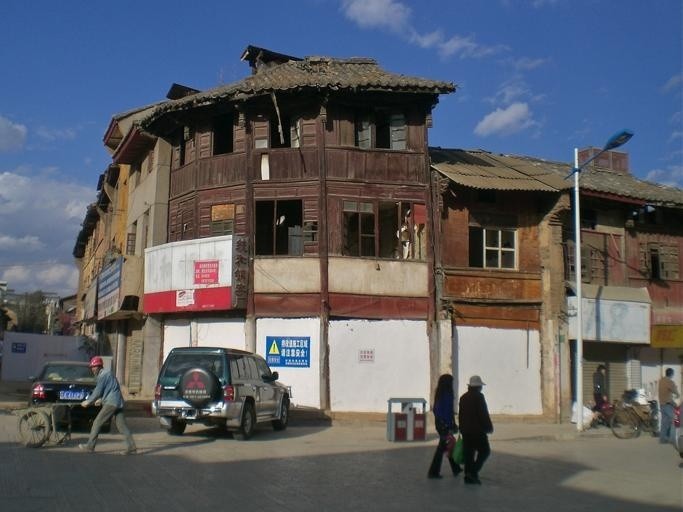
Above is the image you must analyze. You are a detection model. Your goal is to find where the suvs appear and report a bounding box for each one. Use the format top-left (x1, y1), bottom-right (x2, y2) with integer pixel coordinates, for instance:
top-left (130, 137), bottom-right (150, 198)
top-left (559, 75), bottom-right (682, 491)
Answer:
top-left (150, 346), bottom-right (291, 442)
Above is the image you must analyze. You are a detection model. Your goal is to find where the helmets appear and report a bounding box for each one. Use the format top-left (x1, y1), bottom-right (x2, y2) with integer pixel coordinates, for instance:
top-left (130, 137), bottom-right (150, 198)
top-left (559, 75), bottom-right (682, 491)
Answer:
top-left (89, 357), bottom-right (103, 366)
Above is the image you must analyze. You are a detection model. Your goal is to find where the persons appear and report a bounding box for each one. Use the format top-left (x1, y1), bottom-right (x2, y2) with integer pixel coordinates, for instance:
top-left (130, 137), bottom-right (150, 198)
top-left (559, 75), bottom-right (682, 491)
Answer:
top-left (658, 368), bottom-right (679, 444)
top-left (458, 374), bottom-right (493, 484)
top-left (426, 374), bottom-right (464, 478)
top-left (78, 356), bottom-right (137, 457)
top-left (590, 364), bottom-right (608, 412)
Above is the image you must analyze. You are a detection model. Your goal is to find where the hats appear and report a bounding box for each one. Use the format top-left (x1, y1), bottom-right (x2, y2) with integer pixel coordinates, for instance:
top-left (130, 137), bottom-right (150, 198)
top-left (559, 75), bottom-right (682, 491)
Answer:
top-left (469, 375), bottom-right (486, 387)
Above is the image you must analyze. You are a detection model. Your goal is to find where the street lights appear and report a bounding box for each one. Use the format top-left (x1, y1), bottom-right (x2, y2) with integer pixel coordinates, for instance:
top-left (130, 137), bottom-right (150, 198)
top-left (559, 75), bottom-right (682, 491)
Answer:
top-left (563, 129), bottom-right (634, 432)
top-left (41, 298), bottom-right (62, 362)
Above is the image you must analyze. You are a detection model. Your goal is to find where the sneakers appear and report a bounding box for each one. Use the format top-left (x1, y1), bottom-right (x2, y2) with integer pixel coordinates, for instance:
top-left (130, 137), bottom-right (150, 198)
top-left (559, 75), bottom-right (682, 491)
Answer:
top-left (120, 450), bottom-right (137, 456)
top-left (79, 444), bottom-right (94, 453)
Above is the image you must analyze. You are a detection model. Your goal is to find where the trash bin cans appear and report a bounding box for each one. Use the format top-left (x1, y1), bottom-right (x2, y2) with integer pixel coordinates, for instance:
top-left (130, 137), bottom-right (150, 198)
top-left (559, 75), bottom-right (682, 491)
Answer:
top-left (388, 398), bottom-right (426, 442)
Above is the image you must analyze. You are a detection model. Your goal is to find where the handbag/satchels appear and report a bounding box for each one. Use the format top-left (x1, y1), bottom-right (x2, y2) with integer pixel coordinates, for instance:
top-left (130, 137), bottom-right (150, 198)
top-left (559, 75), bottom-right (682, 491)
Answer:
top-left (452, 439), bottom-right (466, 465)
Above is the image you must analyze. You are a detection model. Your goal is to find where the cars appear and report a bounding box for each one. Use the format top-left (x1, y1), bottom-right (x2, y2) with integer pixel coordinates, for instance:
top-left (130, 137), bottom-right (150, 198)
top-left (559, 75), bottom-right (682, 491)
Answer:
top-left (26, 361), bottom-right (112, 432)
top-left (672, 402), bottom-right (683, 458)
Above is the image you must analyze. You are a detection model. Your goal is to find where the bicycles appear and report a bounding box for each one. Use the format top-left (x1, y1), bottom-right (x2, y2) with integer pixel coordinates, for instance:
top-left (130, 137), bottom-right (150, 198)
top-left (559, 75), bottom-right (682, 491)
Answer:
top-left (609, 399), bottom-right (661, 438)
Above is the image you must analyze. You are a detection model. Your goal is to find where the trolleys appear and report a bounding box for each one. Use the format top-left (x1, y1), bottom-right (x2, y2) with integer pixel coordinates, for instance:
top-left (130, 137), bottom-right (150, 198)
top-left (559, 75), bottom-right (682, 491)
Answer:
top-left (9, 399), bottom-right (101, 448)
